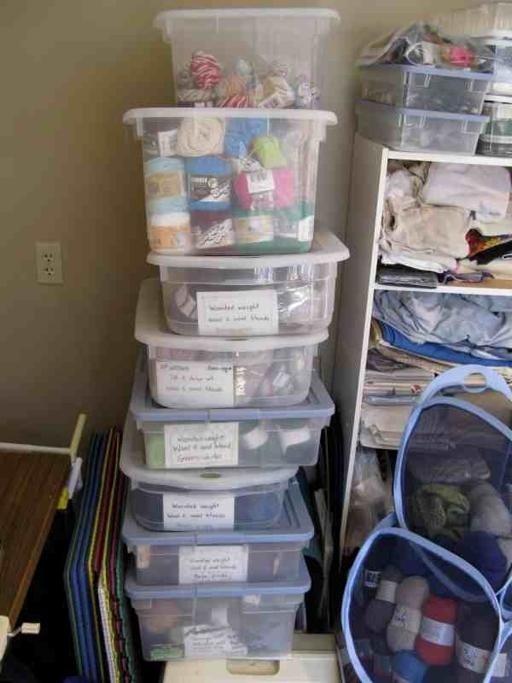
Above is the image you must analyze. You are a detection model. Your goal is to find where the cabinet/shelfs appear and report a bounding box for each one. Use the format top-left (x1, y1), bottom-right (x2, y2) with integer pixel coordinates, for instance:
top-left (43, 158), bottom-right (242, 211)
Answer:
top-left (326, 127), bottom-right (508, 630)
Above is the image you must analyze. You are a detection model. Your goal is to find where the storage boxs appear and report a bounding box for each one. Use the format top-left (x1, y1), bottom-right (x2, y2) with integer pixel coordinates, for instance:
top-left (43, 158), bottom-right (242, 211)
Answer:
top-left (150, 7), bottom-right (342, 108)
top-left (355, 6), bottom-right (511, 159)
top-left (143, 222), bottom-right (352, 335)
top-left (121, 473), bottom-right (316, 588)
top-left (124, 547), bottom-right (313, 662)
top-left (119, 103), bottom-right (341, 255)
top-left (117, 396), bottom-right (300, 534)
top-left (133, 349), bottom-right (333, 471)
top-left (133, 276), bottom-right (329, 408)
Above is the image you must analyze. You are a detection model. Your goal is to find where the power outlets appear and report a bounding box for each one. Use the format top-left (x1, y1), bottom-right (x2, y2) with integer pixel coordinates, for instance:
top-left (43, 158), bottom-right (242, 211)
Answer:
top-left (34, 238), bottom-right (66, 288)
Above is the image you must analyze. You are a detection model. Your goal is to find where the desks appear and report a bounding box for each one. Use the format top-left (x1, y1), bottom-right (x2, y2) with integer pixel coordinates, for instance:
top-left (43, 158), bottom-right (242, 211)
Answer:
top-left (1, 441), bottom-right (73, 683)
